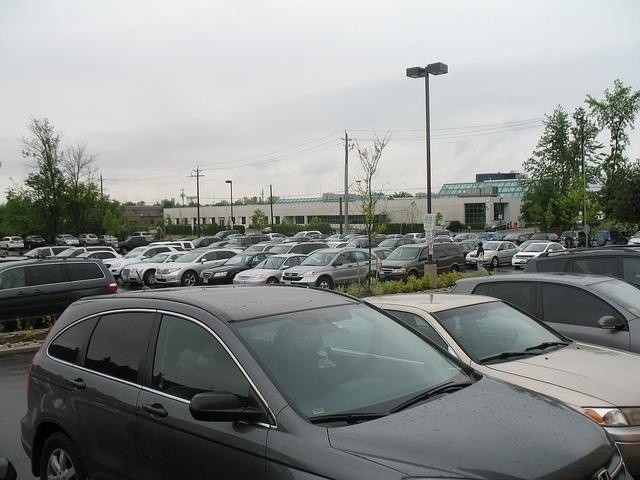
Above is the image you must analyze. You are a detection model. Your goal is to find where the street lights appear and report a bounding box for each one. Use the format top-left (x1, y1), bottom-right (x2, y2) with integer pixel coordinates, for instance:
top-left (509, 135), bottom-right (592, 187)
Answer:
top-left (225, 180), bottom-right (233, 230)
top-left (405, 62), bottom-right (450, 290)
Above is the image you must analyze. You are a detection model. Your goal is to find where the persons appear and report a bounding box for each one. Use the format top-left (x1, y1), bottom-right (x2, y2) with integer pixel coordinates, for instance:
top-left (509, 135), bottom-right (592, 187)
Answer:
top-left (468, 225), bottom-right (471, 233)
top-left (474, 241), bottom-right (485, 270)
top-left (33, 249), bottom-right (46, 259)
